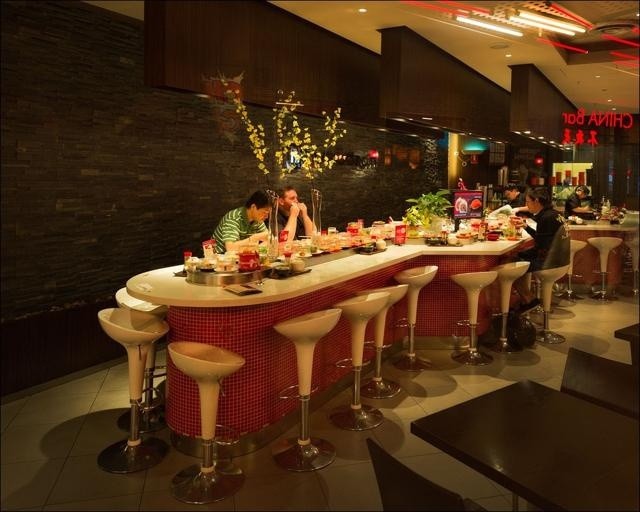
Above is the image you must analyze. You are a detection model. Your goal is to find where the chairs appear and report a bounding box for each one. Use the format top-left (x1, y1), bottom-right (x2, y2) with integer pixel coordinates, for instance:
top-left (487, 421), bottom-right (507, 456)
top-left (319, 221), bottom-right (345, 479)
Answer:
top-left (330, 292), bottom-right (384, 430)
top-left (97, 308), bottom-right (169, 474)
top-left (356, 285), bottom-right (408, 399)
top-left (395, 264), bottom-right (438, 372)
top-left (168, 340), bottom-right (246, 504)
top-left (449, 237), bottom-right (640, 365)
top-left (362, 438), bottom-right (467, 512)
top-left (115, 286), bottom-right (166, 433)
top-left (559, 348), bottom-right (638, 423)
top-left (272, 308), bottom-right (336, 472)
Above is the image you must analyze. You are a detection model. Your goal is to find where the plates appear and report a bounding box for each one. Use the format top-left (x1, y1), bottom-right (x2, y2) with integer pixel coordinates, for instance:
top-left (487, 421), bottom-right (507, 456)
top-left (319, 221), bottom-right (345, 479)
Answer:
top-left (196, 216), bottom-right (523, 279)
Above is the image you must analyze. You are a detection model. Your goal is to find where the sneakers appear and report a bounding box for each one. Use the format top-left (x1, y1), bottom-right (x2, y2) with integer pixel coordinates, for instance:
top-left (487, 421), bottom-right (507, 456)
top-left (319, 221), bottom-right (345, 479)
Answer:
top-left (521, 298), bottom-right (540, 315)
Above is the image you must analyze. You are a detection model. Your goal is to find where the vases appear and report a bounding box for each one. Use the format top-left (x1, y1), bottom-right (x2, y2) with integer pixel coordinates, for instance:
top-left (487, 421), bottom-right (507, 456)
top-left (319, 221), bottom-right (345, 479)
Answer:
top-left (310, 189), bottom-right (322, 251)
top-left (406, 224), bottom-right (419, 239)
top-left (267, 191), bottom-right (277, 262)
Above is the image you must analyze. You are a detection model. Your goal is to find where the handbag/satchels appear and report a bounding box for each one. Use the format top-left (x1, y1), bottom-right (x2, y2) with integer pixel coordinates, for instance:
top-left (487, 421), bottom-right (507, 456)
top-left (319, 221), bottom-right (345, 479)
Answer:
top-left (512, 318), bottom-right (536, 346)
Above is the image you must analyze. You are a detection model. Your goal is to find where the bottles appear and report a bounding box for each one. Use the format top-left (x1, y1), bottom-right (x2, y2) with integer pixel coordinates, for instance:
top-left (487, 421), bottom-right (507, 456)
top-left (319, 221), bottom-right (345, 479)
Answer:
top-left (184, 252), bottom-right (192, 264)
top-left (601, 195), bottom-right (611, 215)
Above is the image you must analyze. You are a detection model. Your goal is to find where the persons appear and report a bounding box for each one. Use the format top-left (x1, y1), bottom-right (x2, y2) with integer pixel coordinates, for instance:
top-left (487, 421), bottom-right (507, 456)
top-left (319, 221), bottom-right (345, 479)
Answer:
top-left (562, 184), bottom-right (599, 220)
top-left (210, 189), bottom-right (275, 253)
top-left (510, 186), bottom-right (567, 315)
top-left (505, 185), bottom-right (530, 217)
top-left (267, 186), bottom-right (317, 242)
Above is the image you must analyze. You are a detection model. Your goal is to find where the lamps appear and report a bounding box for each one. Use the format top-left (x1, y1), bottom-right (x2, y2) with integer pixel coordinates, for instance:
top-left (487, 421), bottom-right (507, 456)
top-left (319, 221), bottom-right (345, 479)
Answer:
top-left (452, 7), bottom-right (585, 36)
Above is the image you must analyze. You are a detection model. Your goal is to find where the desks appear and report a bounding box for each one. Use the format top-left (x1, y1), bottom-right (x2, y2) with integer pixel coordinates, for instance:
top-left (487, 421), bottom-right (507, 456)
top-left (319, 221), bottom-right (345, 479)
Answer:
top-left (409, 376), bottom-right (639, 510)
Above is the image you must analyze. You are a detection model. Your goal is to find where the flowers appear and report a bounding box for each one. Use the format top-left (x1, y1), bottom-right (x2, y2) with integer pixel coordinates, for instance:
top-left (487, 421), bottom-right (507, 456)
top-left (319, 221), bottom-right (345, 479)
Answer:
top-left (292, 108), bottom-right (347, 234)
top-left (402, 207), bottom-right (430, 228)
top-left (218, 77), bottom-right (302, 243)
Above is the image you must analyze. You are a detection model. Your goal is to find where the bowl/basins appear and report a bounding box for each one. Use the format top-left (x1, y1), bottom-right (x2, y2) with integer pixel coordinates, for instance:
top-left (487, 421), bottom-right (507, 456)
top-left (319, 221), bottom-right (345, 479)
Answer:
top-left (531, 170), bottom-right (584, 186)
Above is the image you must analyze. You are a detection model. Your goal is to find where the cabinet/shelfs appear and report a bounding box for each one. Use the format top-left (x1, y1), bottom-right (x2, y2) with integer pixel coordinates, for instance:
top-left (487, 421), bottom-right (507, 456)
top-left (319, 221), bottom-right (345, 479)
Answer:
top-left (550, 162), bottom-right (596, 209)
